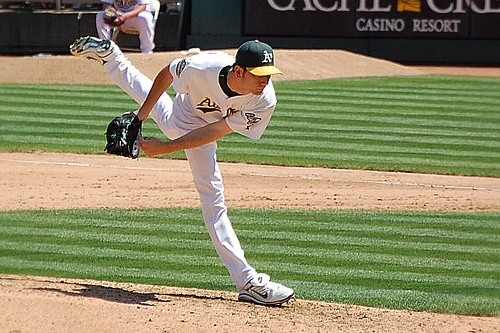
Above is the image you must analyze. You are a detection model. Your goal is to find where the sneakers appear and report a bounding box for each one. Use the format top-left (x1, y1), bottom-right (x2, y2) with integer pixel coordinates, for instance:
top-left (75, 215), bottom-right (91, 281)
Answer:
top-left (238, 272), bottom-right (295, 307)
top-left (69, 35), bottom-right (122, 65)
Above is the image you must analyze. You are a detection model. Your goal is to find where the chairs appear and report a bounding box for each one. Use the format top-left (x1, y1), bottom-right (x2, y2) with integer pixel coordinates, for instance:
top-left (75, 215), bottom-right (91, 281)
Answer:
top-left (111, 0), bottom-right (161, 52)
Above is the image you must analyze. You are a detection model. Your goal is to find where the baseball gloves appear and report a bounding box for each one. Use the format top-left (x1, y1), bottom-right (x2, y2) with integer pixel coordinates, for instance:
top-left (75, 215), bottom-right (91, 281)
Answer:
top-left (104, 112), bottom-right (141, 160)
top-left (103, 7), bottom-right (125, 28)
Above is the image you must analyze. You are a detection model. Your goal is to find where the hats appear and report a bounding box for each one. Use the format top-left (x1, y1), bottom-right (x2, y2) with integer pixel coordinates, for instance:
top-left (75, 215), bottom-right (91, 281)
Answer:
top-left (235, 39), bottom-right (283, 76)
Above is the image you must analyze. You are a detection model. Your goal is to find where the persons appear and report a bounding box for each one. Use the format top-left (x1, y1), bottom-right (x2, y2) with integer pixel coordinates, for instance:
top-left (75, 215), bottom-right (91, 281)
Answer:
top-left (68, 35), bottom-right (296, 308)
top-left (95, 0), bottom-right (161, 55)
